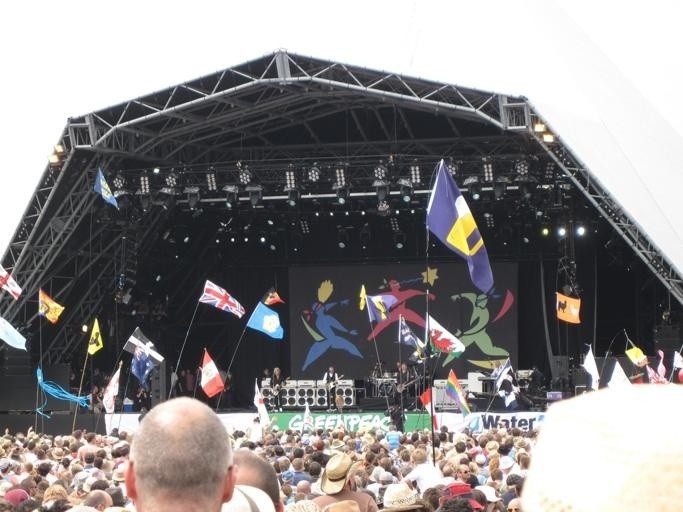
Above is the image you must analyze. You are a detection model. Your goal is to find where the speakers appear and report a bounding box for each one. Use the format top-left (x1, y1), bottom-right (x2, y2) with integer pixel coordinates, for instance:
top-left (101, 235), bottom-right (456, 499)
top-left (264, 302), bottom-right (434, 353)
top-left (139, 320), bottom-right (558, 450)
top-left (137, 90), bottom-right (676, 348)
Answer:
top-left (4, 335), bottom-right (32, 375)
top-left (654, 323), bottom-right (682, 358)
top-left (260, 378), bottom-right (356, 411)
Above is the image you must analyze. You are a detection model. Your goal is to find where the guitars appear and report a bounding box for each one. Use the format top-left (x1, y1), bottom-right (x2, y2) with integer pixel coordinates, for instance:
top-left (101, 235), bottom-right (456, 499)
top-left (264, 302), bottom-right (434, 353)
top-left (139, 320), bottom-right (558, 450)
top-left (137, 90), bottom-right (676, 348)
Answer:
top-left (326, 375), bottom-right (344, 391)
top-left (397, 375), bottom-right (421, 393)
top-left (272, 377), bottom-right (290, 397)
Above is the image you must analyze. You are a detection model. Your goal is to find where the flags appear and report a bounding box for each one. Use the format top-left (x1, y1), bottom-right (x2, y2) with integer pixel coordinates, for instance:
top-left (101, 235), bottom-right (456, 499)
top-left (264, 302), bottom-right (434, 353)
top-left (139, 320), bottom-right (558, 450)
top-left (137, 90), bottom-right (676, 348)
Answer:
top-left (246, 301), bottom-right (284, 340)
top-left (199, 279), bottom-right (246, 320)
top-left (365, 294), bottom-right (398, 322)
top-left (424, 312), bottom-right (466, 359)
top-left (0, 317), bottom-right (27, 352)
top-left (555, 291), bottom-right (582, 324)
top-left (92, 169), bottom-right (121, 209)
top-left (0, 263), bottom-right (22, 301)
top-left (200, 347), bottom-right (224, 398)
top-left (260, 287), bottom-right (285, 305)
top-left (38, 289), bottom-right (65, 324)
top-left (87, 318), bottom-right (104, 355)
top-left (426, 158), bottom-right (495, 294)
top-left (122, 327), bottom-right (165, 389)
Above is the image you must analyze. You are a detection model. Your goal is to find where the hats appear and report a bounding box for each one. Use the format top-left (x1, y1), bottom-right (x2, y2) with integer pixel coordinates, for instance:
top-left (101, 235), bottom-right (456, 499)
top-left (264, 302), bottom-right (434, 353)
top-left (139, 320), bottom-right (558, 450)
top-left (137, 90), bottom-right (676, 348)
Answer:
top-left (321, 453), bottom-right (363, 494)
top-left (112, 469), bottom-right (125, 481)
top-left (51, 447), bottom-right (65, 459)
top-left (476, 454), bottom-right (486, 463)
top-left (220, 486), bottom-right (276, 512)
top-left (500, 456), bottom-right (514, 470)
top-left (54, 436), bottom-right (64, 447)
top-left (516, 437), bottom-right (527, 448)
top-left (330, 439), bottom-right (344, 449)
top-left (475, 485), bottom-right (504, 503)
top-left (430, 448), bottom-right (441, 459)
top-left (5, 489), bottom-right (29, 507)
top-left (377, 484), bottom-right (425, 512)
top-left (486, 441), bottom-right (499, 452)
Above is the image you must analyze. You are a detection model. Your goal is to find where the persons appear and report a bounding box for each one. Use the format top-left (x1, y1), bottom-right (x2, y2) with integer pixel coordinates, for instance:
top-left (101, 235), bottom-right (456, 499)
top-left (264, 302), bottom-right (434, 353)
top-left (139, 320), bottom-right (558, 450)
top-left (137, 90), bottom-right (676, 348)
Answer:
top-left (1, 362), bottom-right (683, 511)
